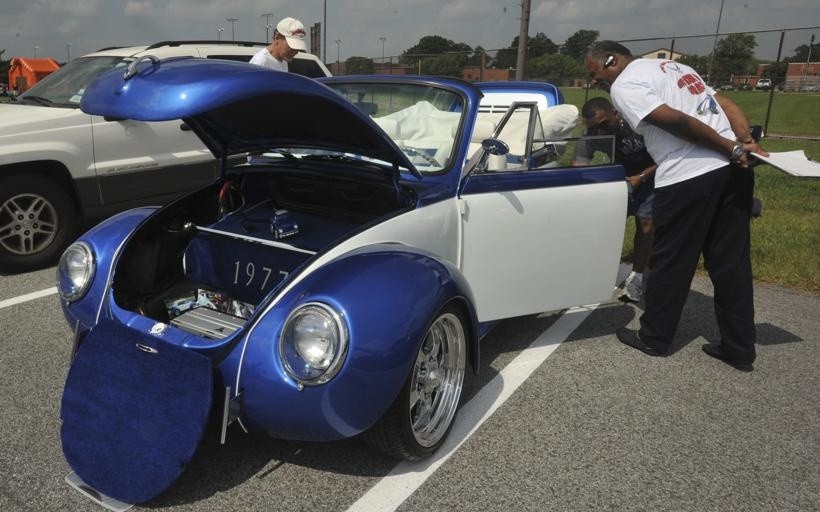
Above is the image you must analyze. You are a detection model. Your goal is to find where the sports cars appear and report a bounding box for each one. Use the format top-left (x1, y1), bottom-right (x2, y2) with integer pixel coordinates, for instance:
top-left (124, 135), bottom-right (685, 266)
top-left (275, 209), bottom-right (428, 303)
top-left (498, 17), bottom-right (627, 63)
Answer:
top-left (55, 54), bottom-right (630, 504)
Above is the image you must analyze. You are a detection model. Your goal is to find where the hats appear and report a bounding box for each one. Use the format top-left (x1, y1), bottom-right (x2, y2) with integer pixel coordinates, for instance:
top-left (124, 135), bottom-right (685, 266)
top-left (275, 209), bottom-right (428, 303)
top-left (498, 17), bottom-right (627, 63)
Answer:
top-left (277, 17), bottom-right (307, 53)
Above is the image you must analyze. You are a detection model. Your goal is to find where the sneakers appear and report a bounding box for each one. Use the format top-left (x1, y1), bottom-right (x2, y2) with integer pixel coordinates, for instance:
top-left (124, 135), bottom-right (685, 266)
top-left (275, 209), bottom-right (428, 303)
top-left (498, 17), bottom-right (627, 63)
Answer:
top-left (616, 326), bottom-right (662, 356)
top-left (617, 272), bottom-right (644, 302)
top-left (702, 343), bottom-right (756, 366)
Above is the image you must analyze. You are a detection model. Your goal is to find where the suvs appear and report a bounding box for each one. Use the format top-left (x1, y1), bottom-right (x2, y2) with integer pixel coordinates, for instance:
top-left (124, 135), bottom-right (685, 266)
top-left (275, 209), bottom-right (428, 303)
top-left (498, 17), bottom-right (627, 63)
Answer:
top-left (0, 39), bottom-right (346, 275)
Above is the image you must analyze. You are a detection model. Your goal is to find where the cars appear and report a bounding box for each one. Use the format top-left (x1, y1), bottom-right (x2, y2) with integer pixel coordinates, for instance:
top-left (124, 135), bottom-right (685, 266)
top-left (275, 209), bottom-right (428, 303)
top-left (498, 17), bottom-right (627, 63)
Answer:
top-left (720, 77), bottom-right (772, 90)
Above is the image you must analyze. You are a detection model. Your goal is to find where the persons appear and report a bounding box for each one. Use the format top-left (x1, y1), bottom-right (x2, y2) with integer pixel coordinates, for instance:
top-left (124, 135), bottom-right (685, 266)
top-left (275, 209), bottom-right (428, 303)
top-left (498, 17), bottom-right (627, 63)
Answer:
top-left (585, 39), bottom-right (769, 368)
top-left (570, 97), bottom-right (657, 302)
top-left (249, 17), bottom-right (306, 73)
top-left (12, 83), bottom-right (18, 97)
top-left (2, 84), bottom-right (6, 93)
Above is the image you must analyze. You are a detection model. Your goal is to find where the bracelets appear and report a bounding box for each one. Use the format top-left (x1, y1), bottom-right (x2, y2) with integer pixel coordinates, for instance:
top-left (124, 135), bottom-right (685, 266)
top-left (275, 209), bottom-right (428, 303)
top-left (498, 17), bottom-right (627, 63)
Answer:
top-left (638, 171), bottom-right (646, 184)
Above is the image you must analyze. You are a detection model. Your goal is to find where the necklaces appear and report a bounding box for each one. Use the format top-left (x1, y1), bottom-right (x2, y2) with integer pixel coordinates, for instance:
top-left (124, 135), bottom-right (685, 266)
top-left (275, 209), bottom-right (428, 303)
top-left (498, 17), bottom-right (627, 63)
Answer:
top-left (619, 119), bottom-right (624, 132)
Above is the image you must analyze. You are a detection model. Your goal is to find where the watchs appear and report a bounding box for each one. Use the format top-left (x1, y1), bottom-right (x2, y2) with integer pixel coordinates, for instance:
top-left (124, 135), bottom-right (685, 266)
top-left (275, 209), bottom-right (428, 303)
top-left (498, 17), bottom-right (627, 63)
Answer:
top-left (728, 141), bottom-right (743, 165)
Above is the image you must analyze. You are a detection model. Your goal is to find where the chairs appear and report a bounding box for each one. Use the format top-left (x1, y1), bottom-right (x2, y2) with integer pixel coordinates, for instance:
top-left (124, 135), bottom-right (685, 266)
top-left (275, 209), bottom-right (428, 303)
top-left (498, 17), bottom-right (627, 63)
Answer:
top-left (371, 111), bottom-right (509, 174)
